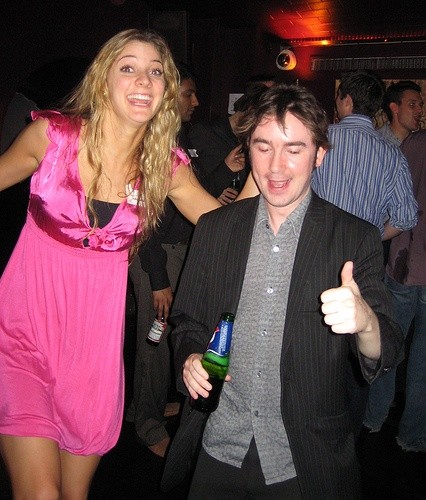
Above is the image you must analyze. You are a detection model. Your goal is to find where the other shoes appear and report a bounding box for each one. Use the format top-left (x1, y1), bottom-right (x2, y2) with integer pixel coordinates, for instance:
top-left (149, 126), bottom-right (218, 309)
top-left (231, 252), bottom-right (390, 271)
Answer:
top-left (163, 403), bottom-right (180, 417)
top-left (138, 420), bottom-right (169, 457)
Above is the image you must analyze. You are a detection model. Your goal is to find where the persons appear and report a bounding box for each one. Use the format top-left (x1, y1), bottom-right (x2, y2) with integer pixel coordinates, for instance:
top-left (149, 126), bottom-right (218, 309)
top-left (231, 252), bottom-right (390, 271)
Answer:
top-left (309, 70), bottom-right (419, 242)
top-left (354, 128), bottom-right (426, 452)
top-left (128, 63), bottom-right (245, 464)
top-left (377, 80), bottom-right (424, 147)
top-left (0, 28), bottom-right (261, 500)
top-left (166, 82), bottom-right (405, 500)
top-left (185, 82), bottom-right (271, 206)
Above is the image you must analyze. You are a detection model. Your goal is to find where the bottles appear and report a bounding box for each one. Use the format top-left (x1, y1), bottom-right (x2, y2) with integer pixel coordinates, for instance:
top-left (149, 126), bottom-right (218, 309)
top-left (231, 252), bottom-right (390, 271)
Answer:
top-left (189, 312), bottom-right (235, 413)
top-left (145, 309), bottom-right (168, 347)
top-left (231, 173), bottom-right (241, 200)
